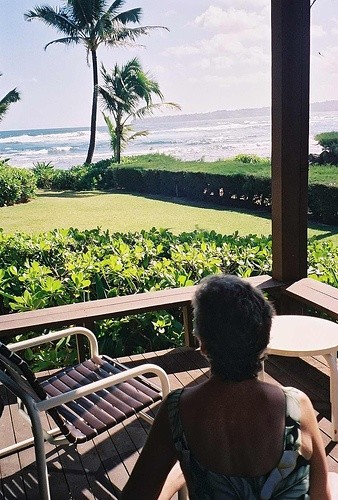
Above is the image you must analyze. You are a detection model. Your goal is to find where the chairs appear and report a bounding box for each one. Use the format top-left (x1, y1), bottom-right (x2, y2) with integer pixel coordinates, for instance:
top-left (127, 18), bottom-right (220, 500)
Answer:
top-left (0, 326), bottom-right (171, 500)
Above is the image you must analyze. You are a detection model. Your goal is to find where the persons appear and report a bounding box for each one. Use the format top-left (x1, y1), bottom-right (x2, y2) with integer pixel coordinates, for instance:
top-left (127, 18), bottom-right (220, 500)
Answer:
top-left (122, 274), bottom-right (335, 500)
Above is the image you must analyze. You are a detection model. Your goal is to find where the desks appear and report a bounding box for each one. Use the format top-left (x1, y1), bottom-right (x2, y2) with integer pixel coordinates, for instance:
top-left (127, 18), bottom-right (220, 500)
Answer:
top-left (264, 310), bottom-right (338, 443)
